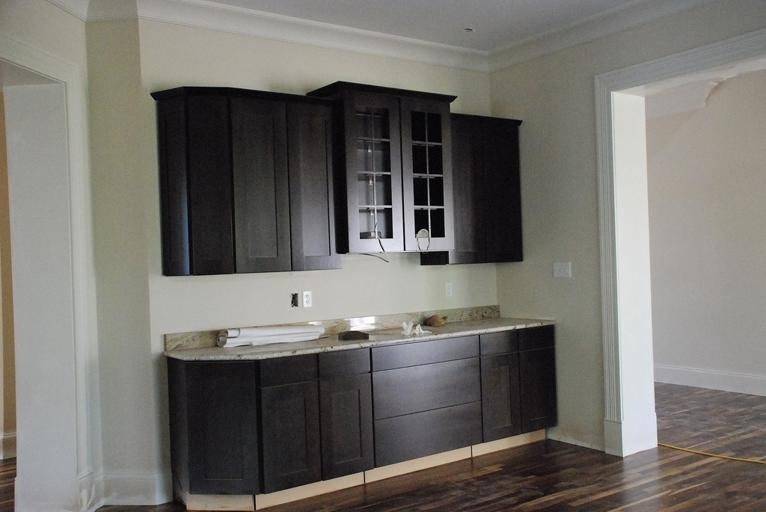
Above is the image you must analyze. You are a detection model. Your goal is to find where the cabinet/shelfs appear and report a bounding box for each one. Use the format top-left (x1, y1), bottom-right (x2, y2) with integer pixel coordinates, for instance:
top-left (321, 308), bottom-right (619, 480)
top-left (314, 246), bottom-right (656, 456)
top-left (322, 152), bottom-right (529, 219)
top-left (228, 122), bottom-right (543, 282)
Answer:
top-left (420, 112), bottom-right (524, 267)
top-left (371, 325), bottom-right (558, 482)
top-left (307, 80), bottom-right (458, 255)
top-left (150, 86), bottom-right (343, 277)
top-left (257, 347), bottom-right (375, 512)
top-left (164, 357), bottom-right (261, 511)
